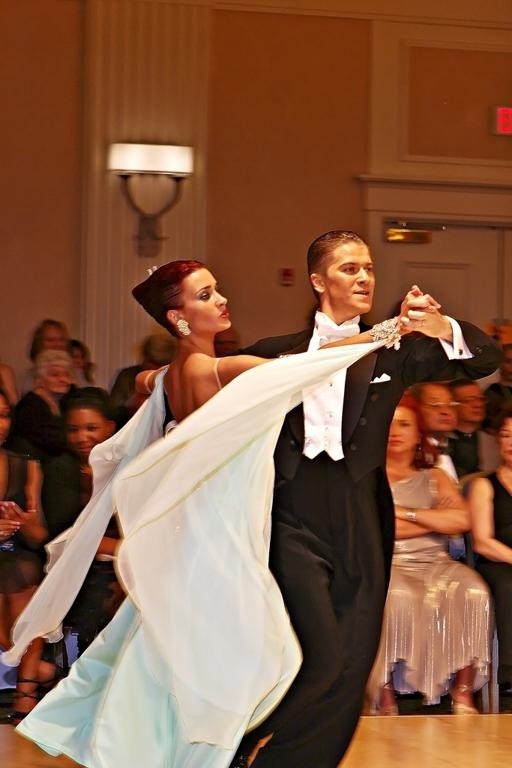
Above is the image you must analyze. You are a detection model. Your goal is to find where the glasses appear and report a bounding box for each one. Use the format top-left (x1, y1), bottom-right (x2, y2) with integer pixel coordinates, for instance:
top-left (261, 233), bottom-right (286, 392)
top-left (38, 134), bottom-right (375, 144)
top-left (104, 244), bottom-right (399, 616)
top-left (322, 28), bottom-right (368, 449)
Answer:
top-left (421, 399), bottom-right (462, 411)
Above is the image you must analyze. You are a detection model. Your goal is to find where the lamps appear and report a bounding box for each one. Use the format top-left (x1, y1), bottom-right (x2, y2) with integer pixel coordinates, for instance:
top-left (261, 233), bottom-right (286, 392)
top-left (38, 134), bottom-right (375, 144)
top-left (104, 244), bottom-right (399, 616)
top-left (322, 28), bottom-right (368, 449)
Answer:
top-left (105, 140), bottom-right (195, 259)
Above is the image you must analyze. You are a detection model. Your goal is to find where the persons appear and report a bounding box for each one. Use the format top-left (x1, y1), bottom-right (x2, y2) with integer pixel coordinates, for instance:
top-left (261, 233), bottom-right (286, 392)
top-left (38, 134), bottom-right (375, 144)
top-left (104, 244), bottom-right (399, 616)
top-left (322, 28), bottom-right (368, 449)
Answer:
top-left (161, 230), bottom-right (506, 767)
top-left (0, 260), bottom-right (440, 767)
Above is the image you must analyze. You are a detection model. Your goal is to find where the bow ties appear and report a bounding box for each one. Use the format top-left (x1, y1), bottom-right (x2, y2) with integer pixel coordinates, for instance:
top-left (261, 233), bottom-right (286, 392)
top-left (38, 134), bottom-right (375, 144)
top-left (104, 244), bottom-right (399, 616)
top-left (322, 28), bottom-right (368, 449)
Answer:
top-left (317, 321), bottom-right (362, 340)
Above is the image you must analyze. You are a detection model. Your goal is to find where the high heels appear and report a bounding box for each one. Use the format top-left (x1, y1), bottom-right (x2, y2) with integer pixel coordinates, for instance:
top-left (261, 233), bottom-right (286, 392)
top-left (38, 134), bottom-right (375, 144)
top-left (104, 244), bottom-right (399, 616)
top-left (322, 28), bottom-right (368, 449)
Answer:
top-left (377, 681), bottom-right (400, 716)
top-left (449, 684), bottom-right (479, 716)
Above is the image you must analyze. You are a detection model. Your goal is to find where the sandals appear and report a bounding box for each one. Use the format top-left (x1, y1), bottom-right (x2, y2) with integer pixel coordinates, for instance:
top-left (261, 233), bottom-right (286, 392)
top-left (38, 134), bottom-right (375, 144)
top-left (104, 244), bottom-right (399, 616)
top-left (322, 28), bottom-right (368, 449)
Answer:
top-left (36, 659), bottom-right (63, 695)
top-left (9, 676), bottom-right (39, 728)
